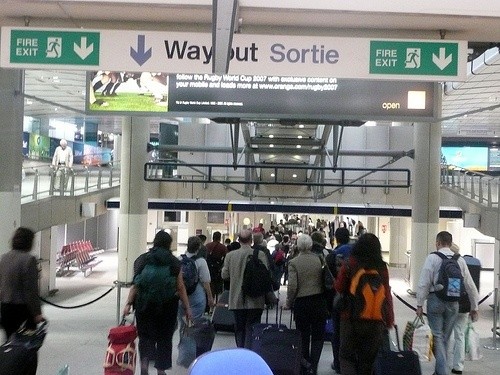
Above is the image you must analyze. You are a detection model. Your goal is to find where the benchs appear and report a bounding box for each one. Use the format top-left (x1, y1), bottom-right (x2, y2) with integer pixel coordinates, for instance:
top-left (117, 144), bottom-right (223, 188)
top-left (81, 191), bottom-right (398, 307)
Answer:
top-left (56, 239), bottom-right (106, 277)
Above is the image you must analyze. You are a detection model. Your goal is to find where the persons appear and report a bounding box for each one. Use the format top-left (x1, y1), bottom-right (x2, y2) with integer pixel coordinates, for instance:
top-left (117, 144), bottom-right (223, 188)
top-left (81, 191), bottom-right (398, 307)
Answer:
top-left (133, 230), bottom-right (192, 362)
top-left (123, 250), bottom-right (180, 375)
top-left (175, 236), bottom-right (213, 368)
top-left (415, 231), bottom-right (480, 375)
top-left (195, 231), bottom-right (241, 301)
top-left (447, 243), bottom-right (470, 375)
top-left (221, 229), bottom-right (276, 350)
top-left (322, 226), bottom-right (395, 375)
top-left (0, 227), bottom-right (43, 375)
top-left (316, 216), bottom-right (364, 248)
top-left (282, 234), bottom-right (326, 375)
top-left (252, 213), bottom-right (334, 304)
top-left (50, 138), bottom-right (73, 190)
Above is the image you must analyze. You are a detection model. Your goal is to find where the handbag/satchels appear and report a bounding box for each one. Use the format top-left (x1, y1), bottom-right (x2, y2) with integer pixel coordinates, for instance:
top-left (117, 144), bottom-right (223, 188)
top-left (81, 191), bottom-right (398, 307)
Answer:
top-left (206, 255), bottom-right (223, 270)
top-left (465, 316), bottom-right (484, 361)
top-left (176, 325), bottom-right (197, 367)
top-left (413, 315), bottom-right (434, 362)
top-left (319, 255), bottom-right (337, 299)
top-left (401, 313), bottom-right (426, 353)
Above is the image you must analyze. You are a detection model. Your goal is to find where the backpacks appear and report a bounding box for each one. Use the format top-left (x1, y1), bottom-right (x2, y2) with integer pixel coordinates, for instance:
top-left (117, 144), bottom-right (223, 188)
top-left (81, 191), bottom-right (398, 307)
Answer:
top-left (429, 251), bottom-right (463, 301)
top-left (180, 253), bottom-right (203, 296)
top-left (344, 251), bottom-right (386, 341)
top-left (133, 248), bottom-right (177, 316)
top-left (242, 248), bottom-right (269, 308)
top-left (275, 250), bottom-right (284, 265)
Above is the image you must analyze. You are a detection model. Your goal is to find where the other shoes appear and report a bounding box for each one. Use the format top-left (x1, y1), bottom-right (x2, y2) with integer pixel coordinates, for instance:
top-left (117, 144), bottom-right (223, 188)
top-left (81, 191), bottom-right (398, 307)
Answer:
top-left (309, 366), bottom-right (316, 375)
top-left (158, 371), bottom-right (167, 375)
top-left (451, 368), bottom-right (463, 374)
top-left (141, 361), bottom-right (150, 375)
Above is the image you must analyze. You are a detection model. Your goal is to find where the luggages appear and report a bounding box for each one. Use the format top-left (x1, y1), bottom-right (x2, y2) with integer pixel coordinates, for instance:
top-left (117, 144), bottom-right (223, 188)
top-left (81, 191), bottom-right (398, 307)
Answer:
top-left (103, 310), bottom-right (138, 374)
top-left (374, 324), bottom-right (421, 375)
top-left (209, 279), bottom-right (238, 334)
top-left (259, 307), bottom-right (303, 375)
top-left (251, 298), bottom-right (288, 351)
top-left (180, 302), bottom-right (217, 366)
top-left (0, 318), bottom-right (47, 375)
